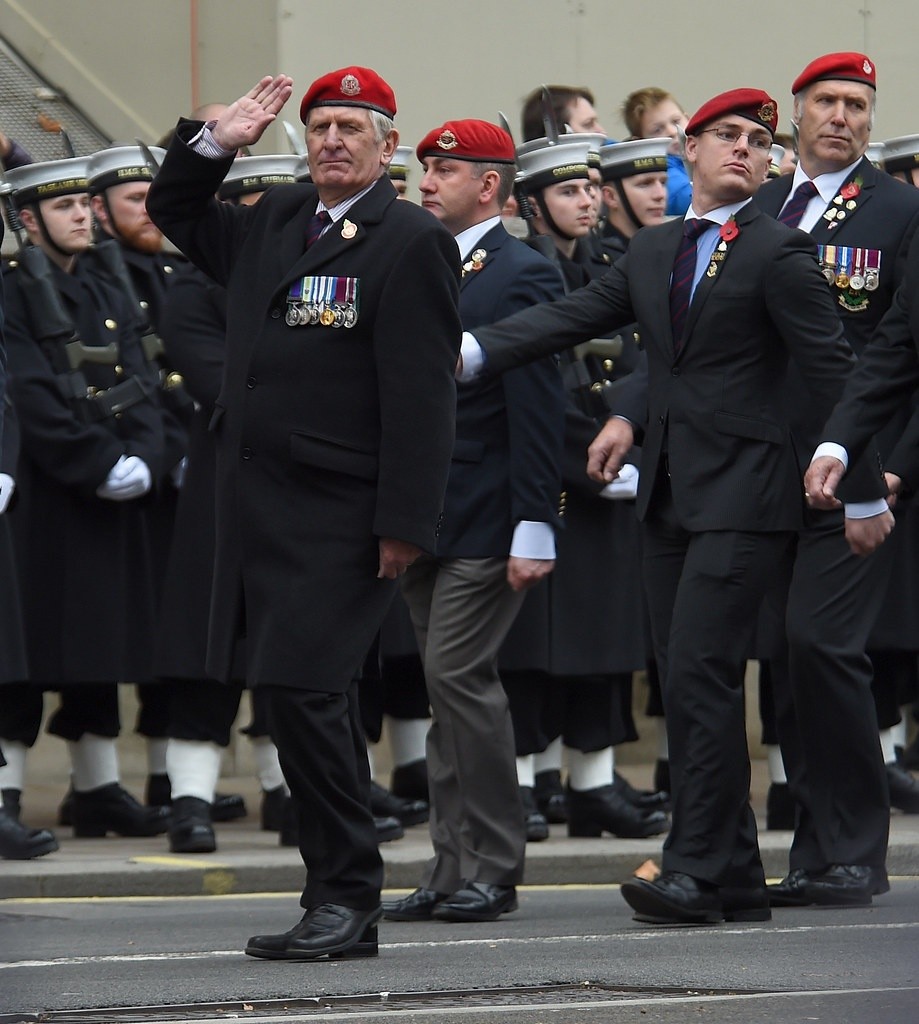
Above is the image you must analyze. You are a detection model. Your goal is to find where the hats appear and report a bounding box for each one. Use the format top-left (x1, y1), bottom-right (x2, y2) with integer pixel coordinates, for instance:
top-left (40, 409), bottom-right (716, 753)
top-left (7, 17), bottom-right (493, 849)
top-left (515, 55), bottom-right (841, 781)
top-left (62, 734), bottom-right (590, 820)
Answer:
top-left (416, 119), bottom-right (517, 164)
top-left (3, 145), bottom-right (167, 210)
top-left (299, 66), bottom-right (398, 125)
top-left (516, 131), bottom-right (674, 192)
top-left (863, 134), bottom-right (919, 174)
top-left (217, 153), bottom-right (310, 200)
top-left (684, 88), bottom-right (778, 134)
top-left (791, 52), bottom-right (876, 94)
top-left (766, 143), bottom-right (785, 179)
top-left (388, 146), bottom-right (415, 180)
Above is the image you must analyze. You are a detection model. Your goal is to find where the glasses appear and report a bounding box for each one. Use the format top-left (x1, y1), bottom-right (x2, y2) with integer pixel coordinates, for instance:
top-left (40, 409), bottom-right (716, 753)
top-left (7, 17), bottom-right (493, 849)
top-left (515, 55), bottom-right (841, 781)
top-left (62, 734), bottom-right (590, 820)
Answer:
top-left (696, 127), bottom-right (775, 149)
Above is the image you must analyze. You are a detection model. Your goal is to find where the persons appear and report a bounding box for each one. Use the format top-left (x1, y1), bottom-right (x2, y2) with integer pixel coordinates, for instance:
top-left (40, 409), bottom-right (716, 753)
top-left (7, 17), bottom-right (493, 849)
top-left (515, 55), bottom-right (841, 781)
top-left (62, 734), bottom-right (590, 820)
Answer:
top-left (0, 87), bottom-right (919, 861)
top-left (457, 87), bottom-right (897, 922)
top-left (377, 121), bottom-right (569, 923)
top-left (587, 53), bottom-right (918, 904)
top-left (145, 67), bottom-right (461, 961)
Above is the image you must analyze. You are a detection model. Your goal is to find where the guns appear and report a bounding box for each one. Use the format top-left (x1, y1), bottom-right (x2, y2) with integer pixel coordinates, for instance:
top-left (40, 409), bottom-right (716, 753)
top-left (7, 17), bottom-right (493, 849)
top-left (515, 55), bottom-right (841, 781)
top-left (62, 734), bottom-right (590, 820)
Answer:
top-left (0, 161), bottom-right (150, 430)
top-left (500, 110), bottom-right (624, 421)
top-left (60, 126), bottom-right (165, 365)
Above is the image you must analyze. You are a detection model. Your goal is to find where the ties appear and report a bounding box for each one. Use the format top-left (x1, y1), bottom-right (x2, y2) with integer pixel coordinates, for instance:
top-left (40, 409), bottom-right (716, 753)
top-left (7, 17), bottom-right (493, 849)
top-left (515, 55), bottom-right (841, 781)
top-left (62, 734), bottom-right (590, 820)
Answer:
top-left (776, 181), bottom-right (820, 230)
top-left (302, 211), bottom-right (333, 254)
top-left (670, 219), bottom-right (719, 358)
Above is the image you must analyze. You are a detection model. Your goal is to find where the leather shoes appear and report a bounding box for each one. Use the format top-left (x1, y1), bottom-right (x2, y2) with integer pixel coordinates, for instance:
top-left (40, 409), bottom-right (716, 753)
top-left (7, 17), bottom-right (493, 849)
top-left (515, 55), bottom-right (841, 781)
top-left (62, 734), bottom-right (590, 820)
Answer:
top-left (372, 816), bottom-right (404, 842)
top-left (392, 759), bottom-right (431, 801)
top-left (535, 783), bottom-right (568, 822)
top-left (245, 905), bottom-right (385, 960)
top-left (886, 762), bottom-right (919, 813)
top-left (259, 787), bottom-right (291, 831)
top-left (145, 775), bottom-right (248, 821)
top-left (520, 786), bottom-right (550, 840)
top-left (0, 815), bottom-right (60, 861)
top-left (622, 872), bottom-right (772, 924)
top-left (57, 788), bottom-right (79, 827)
top-left (1, 789), bottom-right (22, 819)
top-left (613, 769), bottom-right (671, 807)
top-left (172, 796), bottom-right (218, 852)
top-left (767, 782), bottom-right (795, 830)
top-left (767, 864), bottom-right (892, 907)
top-left (370, 781), bottom-right (430, 826)
top-left (76, 784), bottom-right (169, 838)
top-left (281, 797), bottom-right (300, 847)
top-left (567, 786), bottom-right (669, 840)
top-left (655, 759), bottom-right (671, 792)
top-left (380, 882), bottom-right (520, 923)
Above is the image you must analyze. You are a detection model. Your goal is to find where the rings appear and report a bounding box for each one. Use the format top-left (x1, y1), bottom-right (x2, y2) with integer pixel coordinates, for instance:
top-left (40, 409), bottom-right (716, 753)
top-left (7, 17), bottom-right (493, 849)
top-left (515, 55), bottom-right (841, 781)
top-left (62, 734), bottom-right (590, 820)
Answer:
top-left (805, 493), bottom-right (809, 497)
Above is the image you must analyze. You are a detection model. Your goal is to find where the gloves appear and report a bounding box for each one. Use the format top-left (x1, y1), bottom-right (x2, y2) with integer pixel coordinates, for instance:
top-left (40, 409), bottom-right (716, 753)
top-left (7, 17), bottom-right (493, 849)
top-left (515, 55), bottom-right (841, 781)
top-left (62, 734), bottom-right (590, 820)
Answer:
top-left (0, 473), bottom-right (16, 514)
top-left (598, 464), bottom-right (639, 499)
top-left (96, 454), bottom-right (152, 500)
